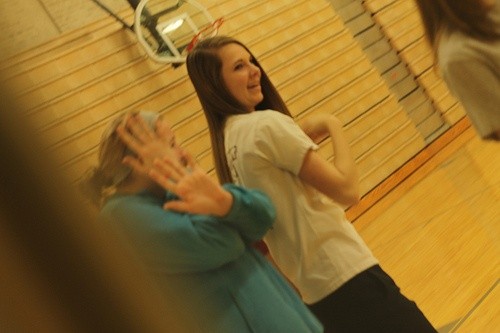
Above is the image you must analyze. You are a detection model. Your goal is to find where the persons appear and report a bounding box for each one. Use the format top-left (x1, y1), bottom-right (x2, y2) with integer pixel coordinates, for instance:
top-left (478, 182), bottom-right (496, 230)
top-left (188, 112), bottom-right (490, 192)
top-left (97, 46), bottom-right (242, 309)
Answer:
top-left (78, 109), bottom-right (325, 332)
top-left (415, 0), bottom-right (500, 145)
top-left (186, 35), bottom-right (439, 333)
